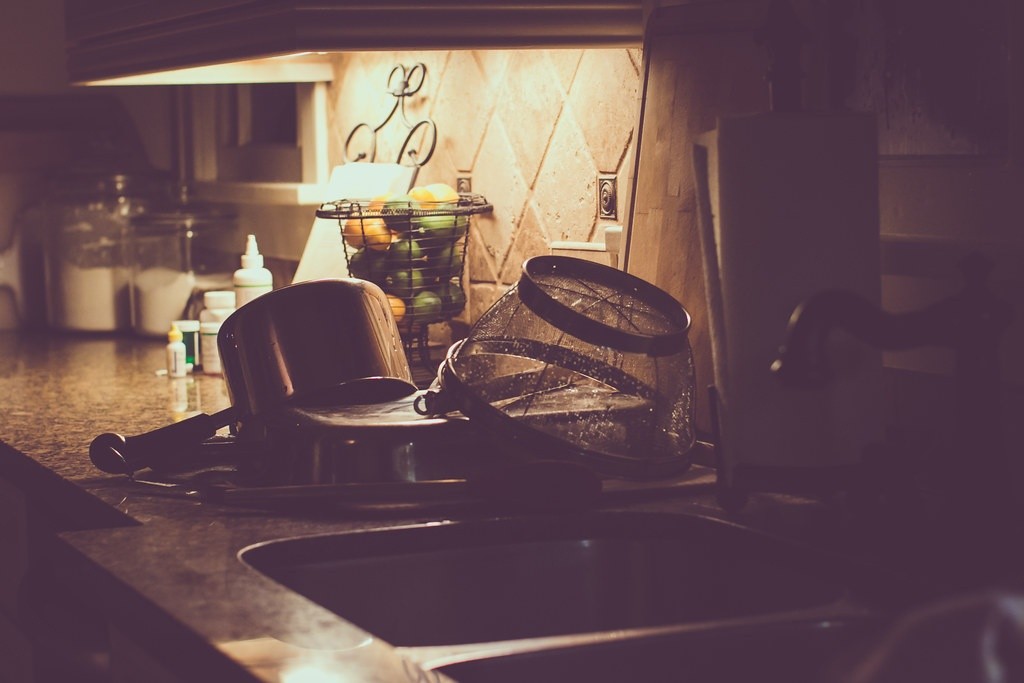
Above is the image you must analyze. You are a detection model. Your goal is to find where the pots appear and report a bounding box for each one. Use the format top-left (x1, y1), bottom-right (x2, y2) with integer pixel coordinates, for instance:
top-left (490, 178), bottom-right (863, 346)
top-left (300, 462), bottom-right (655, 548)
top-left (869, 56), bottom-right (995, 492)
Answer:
top-left (90, 278), bottom-right (420, 475)
top-left (150, 389), bottom-right (657, 484)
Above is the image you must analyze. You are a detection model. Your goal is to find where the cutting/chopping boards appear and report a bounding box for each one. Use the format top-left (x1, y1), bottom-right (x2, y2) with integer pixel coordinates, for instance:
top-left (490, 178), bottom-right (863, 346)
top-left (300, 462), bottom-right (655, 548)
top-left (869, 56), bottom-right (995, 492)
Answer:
top-left (291, 161), bottom-right (418, 279)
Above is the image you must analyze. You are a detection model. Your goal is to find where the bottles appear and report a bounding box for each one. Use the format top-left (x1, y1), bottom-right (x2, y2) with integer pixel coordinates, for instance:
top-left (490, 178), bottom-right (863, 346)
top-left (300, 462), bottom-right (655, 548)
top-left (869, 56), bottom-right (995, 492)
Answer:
top-left (128, 181), bottom-right (238, 336)
top-left (48, 170), bottom-right (151, 332)
top-left (200, 289), bottom-right (237, 374)
top-left (166, 321), bottom-right (186, 379)
top-left (233, 235), bottom-right (274, 309)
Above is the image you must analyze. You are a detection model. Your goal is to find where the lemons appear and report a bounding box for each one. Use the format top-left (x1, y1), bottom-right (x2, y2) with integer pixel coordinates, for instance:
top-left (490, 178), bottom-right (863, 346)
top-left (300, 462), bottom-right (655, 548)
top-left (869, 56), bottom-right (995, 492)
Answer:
top-left (344, 183), bottom-right (466, 323)
